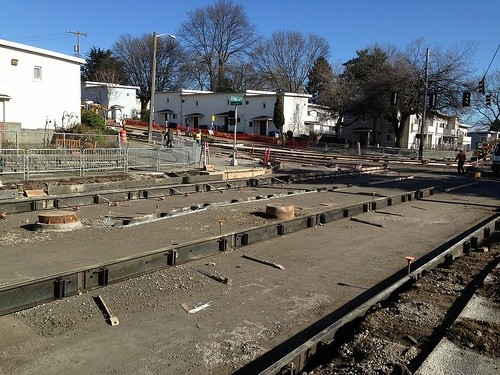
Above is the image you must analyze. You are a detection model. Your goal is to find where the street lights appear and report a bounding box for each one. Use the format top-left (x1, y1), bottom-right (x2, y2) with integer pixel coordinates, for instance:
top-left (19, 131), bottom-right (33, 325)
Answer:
top-left (147, 30), bottom-right (176, 144)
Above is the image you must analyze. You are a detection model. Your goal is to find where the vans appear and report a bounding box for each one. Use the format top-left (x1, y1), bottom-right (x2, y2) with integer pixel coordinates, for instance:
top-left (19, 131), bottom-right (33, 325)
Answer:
top-left (490, 144), bottom-right (500, 176)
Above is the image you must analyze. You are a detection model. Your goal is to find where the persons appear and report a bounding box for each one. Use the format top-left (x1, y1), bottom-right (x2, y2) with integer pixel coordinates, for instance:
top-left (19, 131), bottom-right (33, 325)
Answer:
top-left (316, 134), bottom-right (323, 146)
top-left (455, 148), bottom-right (466, 176)
top-left (194, 129), bottom-right (204, 149)
top-left (186, 121), bottom-right (190, 132)
top-left (164, 120), bottom-right (168, 134)
top-left (282, 133), bottom-right (287, 147)
top-left (164, 128), bottom-right (175, 149)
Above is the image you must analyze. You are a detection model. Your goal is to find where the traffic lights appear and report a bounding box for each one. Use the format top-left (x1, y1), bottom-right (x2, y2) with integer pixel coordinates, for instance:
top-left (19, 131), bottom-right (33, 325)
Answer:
top-left (478, 78), bottom-right (485, 95)
top-left (486, 93), bottom-right (491, 106)
top-left (463, 92), bottom-right (470, 106)
